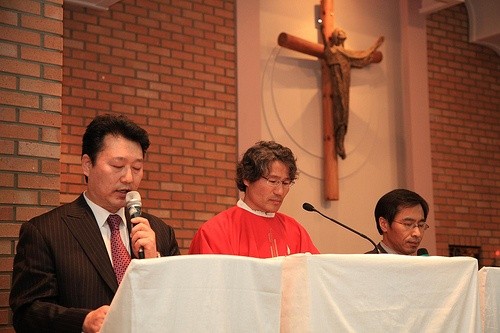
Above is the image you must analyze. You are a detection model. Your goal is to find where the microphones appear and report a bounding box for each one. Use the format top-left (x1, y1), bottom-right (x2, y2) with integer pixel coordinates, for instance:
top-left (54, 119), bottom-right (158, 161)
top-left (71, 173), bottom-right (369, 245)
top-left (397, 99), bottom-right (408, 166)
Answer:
top-left (125, 190), bottom-right (145, 259)
top-left (303, 203), bottom-right (381, 254)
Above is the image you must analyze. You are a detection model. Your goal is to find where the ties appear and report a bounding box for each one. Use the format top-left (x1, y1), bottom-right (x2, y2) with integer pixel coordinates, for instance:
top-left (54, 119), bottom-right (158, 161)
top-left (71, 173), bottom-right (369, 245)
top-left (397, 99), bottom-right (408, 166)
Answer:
top-left (106, 213), bottom-right (130, 287)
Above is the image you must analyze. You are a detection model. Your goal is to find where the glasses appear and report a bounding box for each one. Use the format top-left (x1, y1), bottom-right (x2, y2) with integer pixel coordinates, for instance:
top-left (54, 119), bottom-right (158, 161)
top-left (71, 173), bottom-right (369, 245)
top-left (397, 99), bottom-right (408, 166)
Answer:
top-left (261, 174), bottom-right (295, 189)
top-left (392, 219), bottom-right (430, 232)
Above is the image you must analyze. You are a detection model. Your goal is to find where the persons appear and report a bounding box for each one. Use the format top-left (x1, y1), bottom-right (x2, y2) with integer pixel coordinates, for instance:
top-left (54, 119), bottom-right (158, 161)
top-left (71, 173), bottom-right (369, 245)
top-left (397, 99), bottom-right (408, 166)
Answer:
top-left (364, 189), bottom-right (429, 255)
top-left (9, 113), bottom-right (182, 333)
top-left (187, 139), bottom-right (321, 259)
top-left (320, 5), bottom-right (386, 160)
